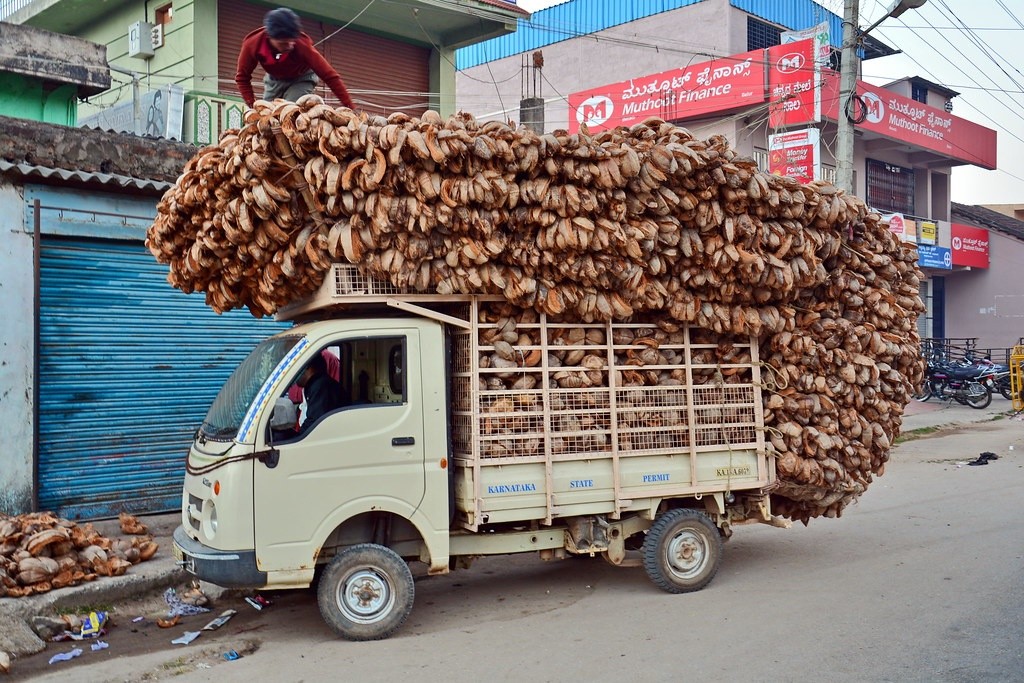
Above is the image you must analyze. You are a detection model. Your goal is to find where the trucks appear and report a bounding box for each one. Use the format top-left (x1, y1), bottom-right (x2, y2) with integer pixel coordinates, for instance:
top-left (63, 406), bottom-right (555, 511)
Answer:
top-left (173, 261), bottom-right (793, 643)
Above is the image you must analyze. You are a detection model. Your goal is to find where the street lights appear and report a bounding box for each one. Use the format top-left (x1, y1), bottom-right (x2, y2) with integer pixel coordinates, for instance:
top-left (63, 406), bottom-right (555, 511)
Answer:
top-left (835, 0), bottom-right (928, 194)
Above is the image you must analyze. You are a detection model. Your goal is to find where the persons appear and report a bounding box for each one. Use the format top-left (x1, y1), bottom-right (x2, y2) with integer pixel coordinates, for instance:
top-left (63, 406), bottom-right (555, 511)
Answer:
top-left (265, 339), bottom-right (354, 445)
top-left (235, 7), bottom-right (358, 113)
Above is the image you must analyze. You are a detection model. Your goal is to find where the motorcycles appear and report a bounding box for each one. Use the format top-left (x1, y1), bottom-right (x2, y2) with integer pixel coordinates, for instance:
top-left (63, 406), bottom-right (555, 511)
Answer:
top-left (911, 341), bottom-right (1024, 410)
